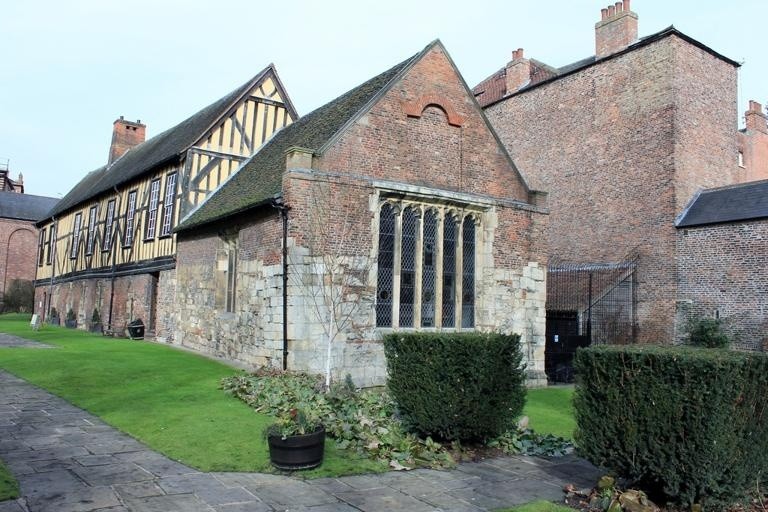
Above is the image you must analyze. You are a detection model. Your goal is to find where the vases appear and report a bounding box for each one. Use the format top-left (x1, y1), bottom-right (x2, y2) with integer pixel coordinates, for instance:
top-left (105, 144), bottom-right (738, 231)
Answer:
top-left (268, 425), bottom-right (324, 470)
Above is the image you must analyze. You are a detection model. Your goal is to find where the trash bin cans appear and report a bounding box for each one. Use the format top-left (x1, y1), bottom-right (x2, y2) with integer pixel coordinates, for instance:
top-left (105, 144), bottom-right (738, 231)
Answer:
top-left (127, 318), bottom-right (144, 339)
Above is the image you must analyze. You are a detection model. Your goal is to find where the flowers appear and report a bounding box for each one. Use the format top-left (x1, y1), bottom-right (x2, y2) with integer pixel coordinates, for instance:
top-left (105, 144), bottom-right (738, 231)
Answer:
top-left (260, 408), bottom-right (325, 445)
